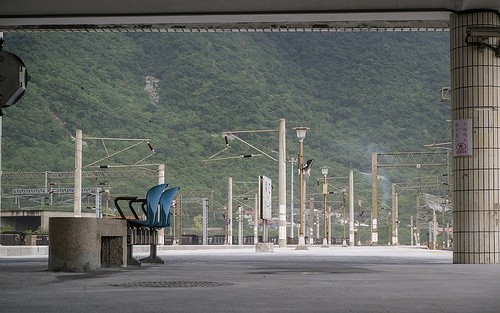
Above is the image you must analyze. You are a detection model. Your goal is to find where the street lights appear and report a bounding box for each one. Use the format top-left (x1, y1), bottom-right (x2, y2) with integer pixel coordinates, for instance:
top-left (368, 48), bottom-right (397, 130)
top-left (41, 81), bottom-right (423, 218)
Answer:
top-left (104, 187), bottom-right (111, 219)
top-left (172, 200), bottom-right (176, 244)
top-left (318, 165), bottom-right (330, 248)
top-left (340, 186), bottom-right (349, 247)
top-left (293, 126), bottom-right (311, 251)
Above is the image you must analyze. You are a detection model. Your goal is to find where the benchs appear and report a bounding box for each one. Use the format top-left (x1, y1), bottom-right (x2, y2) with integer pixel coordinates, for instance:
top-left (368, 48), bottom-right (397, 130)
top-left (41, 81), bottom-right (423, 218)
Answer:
top-left (47, 217), bottom-right (128, 273)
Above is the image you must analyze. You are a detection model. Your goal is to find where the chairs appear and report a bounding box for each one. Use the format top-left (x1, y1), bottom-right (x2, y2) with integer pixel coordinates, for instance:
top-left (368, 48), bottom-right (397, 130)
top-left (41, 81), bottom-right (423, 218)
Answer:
top-left (129, 186), bottom-right (181, 265)
top-left (114, 183), bottom-right (168, 268)
